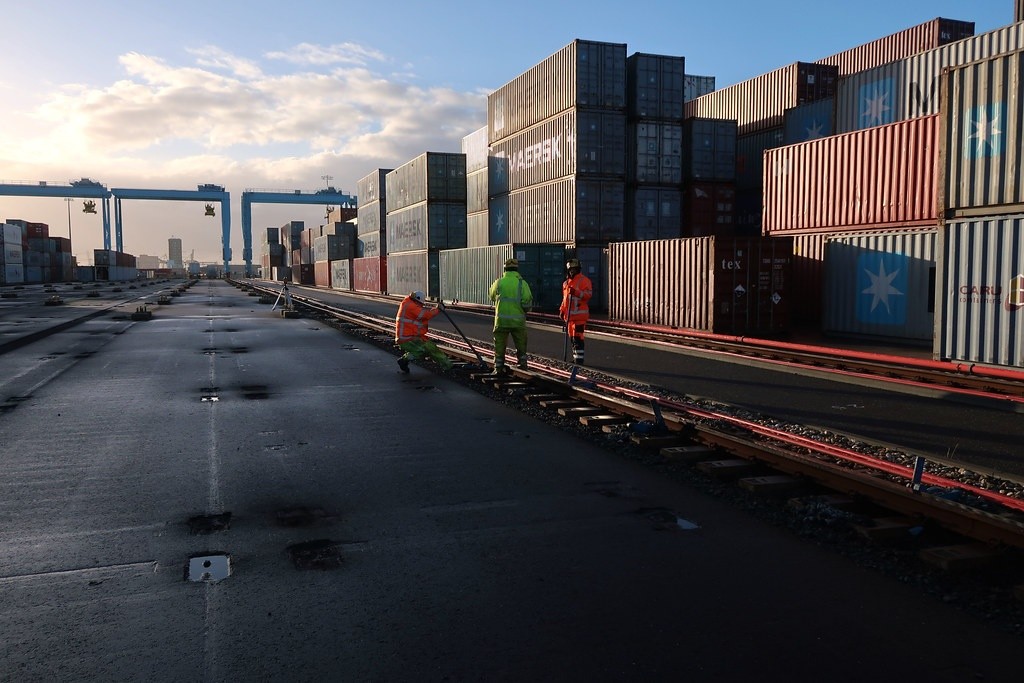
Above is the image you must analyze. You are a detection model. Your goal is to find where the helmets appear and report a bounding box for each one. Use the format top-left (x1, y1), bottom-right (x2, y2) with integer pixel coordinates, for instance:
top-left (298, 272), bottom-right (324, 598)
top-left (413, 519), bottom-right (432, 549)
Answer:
top-left (565, 258), bottom-right (581, 270)
top-left (504, 259), bottom-right (518, 269)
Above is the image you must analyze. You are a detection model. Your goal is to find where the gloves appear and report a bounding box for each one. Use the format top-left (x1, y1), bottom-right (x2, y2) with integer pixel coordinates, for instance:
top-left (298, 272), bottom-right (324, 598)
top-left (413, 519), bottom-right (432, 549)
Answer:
top-left (568, 286), bottom-right (575, 294)
top-left (436, 302), bottom-right (444, 310)
top-left (559, 311), bottom-right (564, 321)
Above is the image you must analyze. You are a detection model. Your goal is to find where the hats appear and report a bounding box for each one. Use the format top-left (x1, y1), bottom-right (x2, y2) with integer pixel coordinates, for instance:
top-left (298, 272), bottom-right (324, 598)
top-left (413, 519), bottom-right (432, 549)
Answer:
top-left (412, 291), bottom-right (426, 304)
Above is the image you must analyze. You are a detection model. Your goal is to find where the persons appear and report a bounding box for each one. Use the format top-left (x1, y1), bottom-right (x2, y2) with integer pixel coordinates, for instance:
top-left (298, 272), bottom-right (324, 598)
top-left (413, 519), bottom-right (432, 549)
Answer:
top-left (558, 258), bottom-right (592, 366)
top-left (394, 290), bottom-right (465, 374)
top-left (488, 258), bottom-right (533, 374)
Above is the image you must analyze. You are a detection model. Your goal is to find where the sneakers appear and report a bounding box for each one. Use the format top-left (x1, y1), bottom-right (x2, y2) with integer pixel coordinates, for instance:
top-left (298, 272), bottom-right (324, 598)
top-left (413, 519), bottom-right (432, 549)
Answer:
top-left (396, 356), bottom-right (410, 373)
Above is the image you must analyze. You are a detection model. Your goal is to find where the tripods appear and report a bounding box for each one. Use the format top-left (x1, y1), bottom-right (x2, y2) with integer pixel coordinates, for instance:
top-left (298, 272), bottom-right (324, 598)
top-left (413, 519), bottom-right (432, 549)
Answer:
top-left (272, 284), bottom-right (292, 310)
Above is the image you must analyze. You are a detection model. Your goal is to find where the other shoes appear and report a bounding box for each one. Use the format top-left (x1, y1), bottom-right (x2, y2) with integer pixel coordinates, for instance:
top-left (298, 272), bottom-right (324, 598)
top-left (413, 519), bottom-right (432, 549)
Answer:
top-left (491, 369), bottom-right (502, 376)
top-left (516, 362), bottom-right (528, 370)
